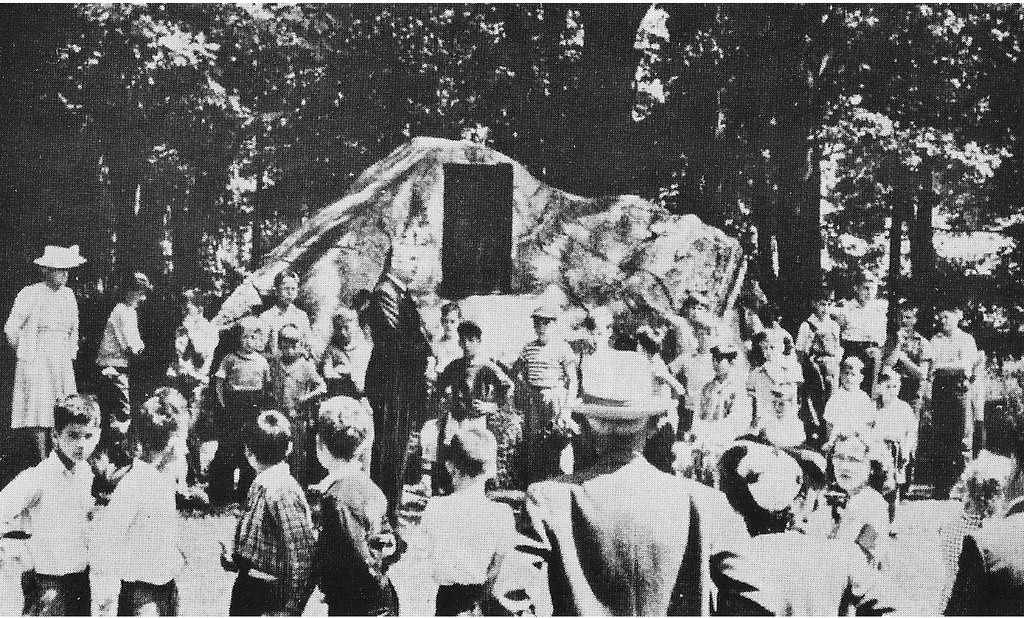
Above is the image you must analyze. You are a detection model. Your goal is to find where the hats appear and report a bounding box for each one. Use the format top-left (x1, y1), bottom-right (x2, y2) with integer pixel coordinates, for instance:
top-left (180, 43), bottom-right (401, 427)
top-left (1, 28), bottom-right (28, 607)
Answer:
top-left (564, 352), bottom-right (680, 417)
top-left (529, 302), bottom-right (559, 322)
top-left (34, 246), bottom-right (90, 267)
top-left (117, 273), bottom-right (157, 293)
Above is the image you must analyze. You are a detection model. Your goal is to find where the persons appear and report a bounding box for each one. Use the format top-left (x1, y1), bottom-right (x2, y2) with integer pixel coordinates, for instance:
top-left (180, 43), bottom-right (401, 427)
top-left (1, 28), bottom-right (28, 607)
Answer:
top-left (303, 394), bottom-right (399, 617)
top-left (707, 438), bottom-right (902, 617)
top-left (2, 244), bottom-right (988, 535)
top-left (217, 409), bottom-right (316, 617)
top-left (98, 386), bottom-right (189, 617)
top-left (939, 437), bottom-right (1024, 617)
top-left (416, 428), bottom-right (517, 617)
top-left (0, 393), bottom-right (101, 616)
top-left (473, 346), bottom-right (751, 617)
top-left (939, 450), bottom-right (1013, 585)
top-left (824, 430), bottom-right (897, 578)
